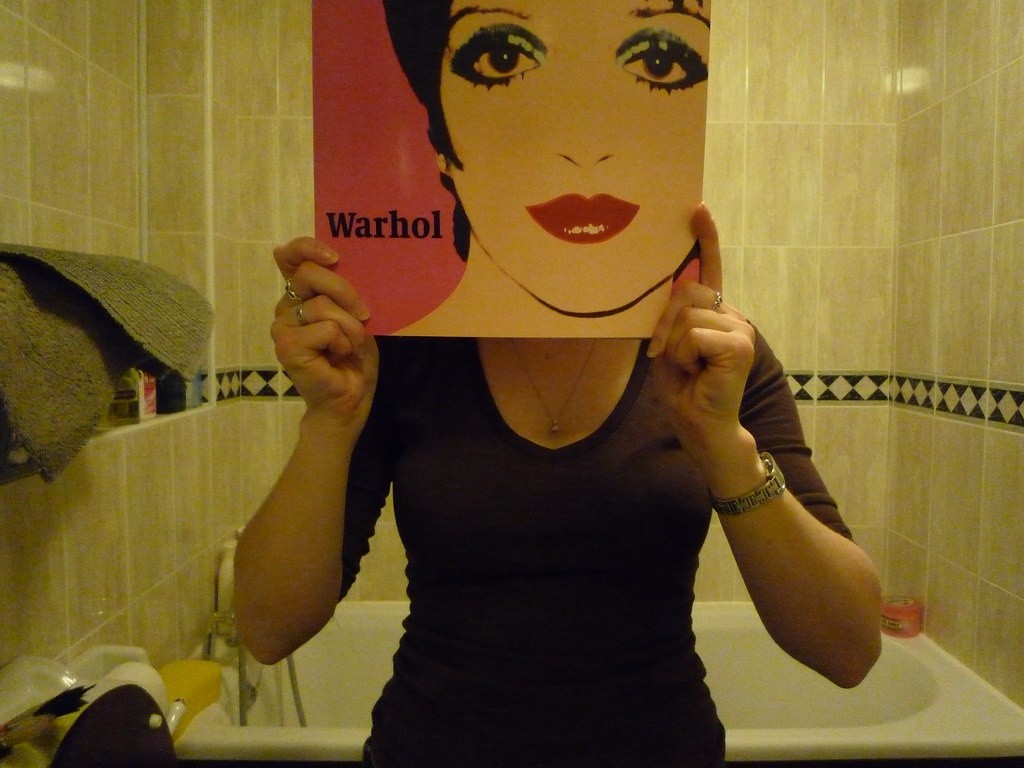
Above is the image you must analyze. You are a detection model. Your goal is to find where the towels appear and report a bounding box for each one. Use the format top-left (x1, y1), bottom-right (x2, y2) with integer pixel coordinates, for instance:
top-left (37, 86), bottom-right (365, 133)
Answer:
top-left (0, 241), bottom-right (215, 485)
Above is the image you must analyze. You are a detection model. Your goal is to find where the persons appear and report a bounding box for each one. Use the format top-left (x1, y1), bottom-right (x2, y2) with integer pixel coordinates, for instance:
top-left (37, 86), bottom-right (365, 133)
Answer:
top-left (230, 202), bottom-right (883, 767)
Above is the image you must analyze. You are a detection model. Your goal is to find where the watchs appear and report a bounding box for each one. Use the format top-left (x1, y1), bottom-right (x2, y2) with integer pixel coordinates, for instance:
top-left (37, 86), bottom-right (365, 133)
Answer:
top-left (708, 448), bottom-right (787, 516)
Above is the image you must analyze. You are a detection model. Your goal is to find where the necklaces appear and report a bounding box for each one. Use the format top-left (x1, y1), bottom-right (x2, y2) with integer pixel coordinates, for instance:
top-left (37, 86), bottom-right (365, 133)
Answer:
top-left (509, 337), bottom-right (596, 436)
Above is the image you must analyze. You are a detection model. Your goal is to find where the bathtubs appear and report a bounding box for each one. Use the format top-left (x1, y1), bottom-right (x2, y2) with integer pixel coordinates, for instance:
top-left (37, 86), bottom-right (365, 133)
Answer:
top-left (151, 594), bottom-right (1023, 759)
